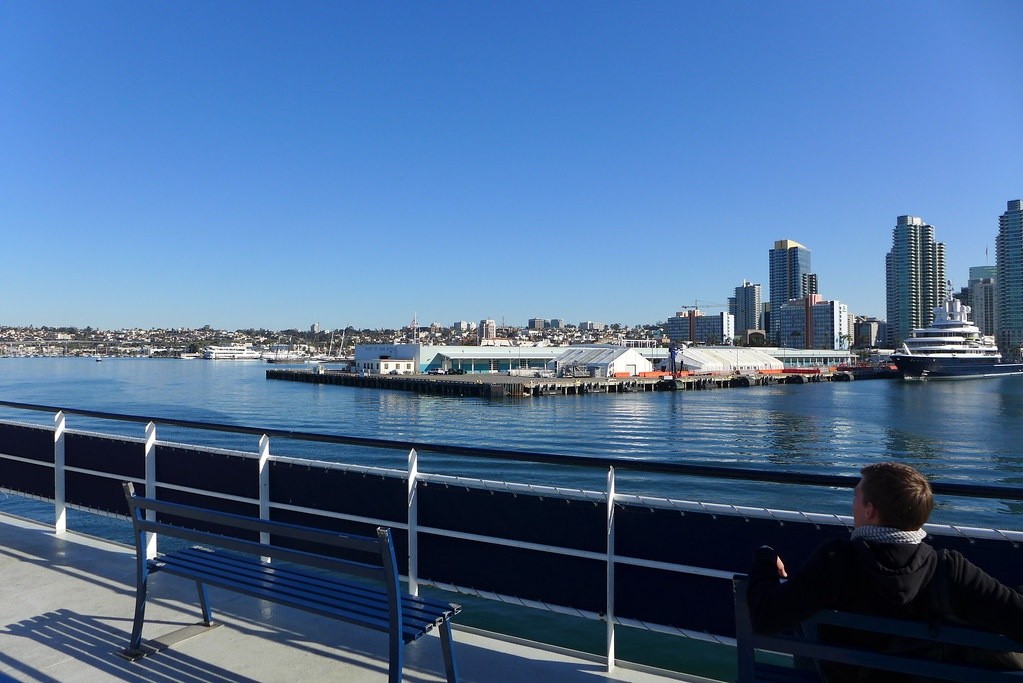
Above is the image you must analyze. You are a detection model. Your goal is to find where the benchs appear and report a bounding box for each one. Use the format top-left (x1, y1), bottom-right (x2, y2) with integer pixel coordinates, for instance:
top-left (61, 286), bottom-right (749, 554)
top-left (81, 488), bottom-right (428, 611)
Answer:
top-left (122, 482), bottom-right (464, 683)
top-left (732, 574), bottom-right (1023, 683)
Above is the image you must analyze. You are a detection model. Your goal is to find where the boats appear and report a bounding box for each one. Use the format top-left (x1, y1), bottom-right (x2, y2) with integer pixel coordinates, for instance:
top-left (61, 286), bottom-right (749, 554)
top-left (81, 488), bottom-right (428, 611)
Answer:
top-left (203, 342), bottom-right (261, 360)
top-left (889, 278), bottom-right (1023, 377)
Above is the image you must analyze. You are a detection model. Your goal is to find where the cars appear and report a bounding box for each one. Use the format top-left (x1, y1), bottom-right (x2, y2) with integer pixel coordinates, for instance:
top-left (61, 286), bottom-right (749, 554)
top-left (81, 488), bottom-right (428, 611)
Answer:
top-left (428, 367), bottom-right (445, 375)
top-left (390, 369), bottom-right (404, 375)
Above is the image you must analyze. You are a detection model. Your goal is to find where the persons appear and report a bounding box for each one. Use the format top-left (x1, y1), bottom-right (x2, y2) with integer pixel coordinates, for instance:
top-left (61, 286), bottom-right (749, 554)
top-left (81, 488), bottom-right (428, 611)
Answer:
top-left (746, 462), bottom-right (1023, 683)
